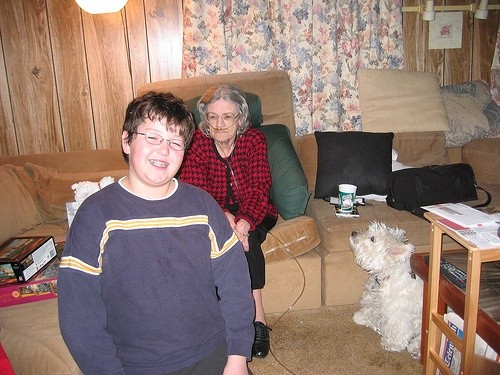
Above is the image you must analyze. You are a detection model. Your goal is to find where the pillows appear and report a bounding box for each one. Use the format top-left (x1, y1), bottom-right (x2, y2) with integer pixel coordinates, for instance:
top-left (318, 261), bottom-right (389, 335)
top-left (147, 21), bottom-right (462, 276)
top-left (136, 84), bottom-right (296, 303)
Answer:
top-left (138, 69), bottom-right (297, 142)
top-left (356, 67), bottom-right (451, 134)
top-left (184, 92), bottom-right (264, 129)
top-left (314, 130), bottom-right (395, 198)
top-left (440, 80), bottom-right (500, 148)
top-left (256, 123), bottom-right (312, 220)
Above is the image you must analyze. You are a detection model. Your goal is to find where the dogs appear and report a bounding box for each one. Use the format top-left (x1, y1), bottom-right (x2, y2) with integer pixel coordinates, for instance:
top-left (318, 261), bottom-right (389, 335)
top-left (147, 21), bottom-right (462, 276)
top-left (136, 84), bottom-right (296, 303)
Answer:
top-left (349, 221), bottom-right (454, 360)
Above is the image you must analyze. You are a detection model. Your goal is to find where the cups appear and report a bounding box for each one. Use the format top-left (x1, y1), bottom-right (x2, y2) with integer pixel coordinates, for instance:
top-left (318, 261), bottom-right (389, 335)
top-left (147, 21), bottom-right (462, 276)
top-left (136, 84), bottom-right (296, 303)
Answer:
top-left (339, 184), bottom-right (357, 212)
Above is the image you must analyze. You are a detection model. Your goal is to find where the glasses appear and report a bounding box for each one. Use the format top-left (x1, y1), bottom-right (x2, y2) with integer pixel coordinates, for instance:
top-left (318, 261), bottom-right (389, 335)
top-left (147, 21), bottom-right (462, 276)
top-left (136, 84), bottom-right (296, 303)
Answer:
top-left (126, 131), bottom-right (188, 151)
top-left (204, 110), bottom-right (241, 122)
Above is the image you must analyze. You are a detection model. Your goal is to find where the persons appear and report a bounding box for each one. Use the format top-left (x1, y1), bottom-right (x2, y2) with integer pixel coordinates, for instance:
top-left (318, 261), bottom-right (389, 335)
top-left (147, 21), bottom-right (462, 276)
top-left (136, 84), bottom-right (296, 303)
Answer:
top-left (58, 92), bottom-right (255, 375)
top-left (178, 83), bottom-right (279, 358)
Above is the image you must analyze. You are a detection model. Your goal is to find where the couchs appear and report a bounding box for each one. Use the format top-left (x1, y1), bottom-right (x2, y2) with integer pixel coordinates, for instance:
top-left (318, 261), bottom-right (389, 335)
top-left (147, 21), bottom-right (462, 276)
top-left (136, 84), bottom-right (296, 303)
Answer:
top-left (0, 128), bottom-right (500, 375)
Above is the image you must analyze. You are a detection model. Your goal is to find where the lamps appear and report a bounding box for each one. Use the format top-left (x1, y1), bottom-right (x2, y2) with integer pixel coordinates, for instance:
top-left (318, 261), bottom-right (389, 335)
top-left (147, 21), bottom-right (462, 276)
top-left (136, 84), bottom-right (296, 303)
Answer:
top-left (474, 0), bottom-right (490, 19)
top-left (422, 0), bottom-right (436, 21)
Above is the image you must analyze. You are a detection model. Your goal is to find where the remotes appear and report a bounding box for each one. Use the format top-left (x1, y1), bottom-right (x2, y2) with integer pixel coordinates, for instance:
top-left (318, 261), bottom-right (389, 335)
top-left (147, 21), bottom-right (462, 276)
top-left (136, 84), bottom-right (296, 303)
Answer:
top-left (425, 255), bottom-right (467, 288)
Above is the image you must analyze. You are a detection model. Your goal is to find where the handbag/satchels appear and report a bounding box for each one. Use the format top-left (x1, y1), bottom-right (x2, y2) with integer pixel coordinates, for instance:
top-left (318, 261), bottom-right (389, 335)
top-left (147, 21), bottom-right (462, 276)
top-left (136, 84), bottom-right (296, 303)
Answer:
top-left (384, 161), bottom-right (491, 225)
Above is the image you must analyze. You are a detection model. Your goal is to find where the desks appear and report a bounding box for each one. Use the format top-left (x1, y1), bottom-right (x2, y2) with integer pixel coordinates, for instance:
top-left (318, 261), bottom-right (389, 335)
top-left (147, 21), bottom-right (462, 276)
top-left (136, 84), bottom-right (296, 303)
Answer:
top-left (410, 248), bottom-right (500, 375)
top-left (424, 205), bottom-right (500, 375)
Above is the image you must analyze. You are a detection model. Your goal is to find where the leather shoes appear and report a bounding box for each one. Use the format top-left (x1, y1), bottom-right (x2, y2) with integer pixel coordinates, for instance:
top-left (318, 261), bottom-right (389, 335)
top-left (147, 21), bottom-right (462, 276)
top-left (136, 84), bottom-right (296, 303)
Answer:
top-left (251, 321), bottom-right (271, 358)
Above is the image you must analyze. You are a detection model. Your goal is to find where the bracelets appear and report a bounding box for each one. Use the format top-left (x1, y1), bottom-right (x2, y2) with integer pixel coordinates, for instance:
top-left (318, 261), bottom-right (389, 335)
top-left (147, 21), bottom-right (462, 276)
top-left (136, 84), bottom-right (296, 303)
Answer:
top-left (234, 226), bottom-right (250, 237)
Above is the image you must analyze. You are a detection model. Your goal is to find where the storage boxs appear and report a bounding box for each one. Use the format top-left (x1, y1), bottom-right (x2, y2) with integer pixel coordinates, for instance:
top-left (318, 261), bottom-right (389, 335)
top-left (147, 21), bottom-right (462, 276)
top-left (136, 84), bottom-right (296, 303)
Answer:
top-left (0, 240), bottom-right (66, 308)
top-left (0, 236), bottom-right (57, 286)
